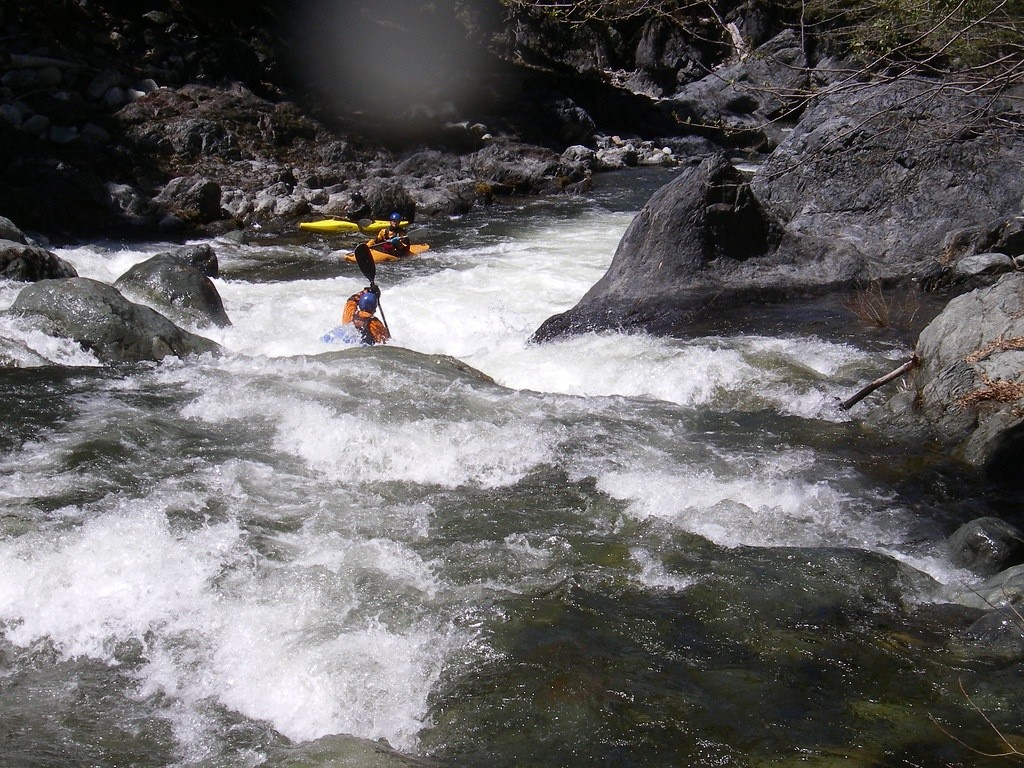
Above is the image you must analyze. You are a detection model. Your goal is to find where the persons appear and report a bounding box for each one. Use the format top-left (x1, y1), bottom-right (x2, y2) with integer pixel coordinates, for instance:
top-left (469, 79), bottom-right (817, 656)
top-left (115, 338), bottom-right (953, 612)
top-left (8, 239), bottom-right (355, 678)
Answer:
top-left (341, 286), bottom-right (389, 343)
top-left (366, 213), bottom-right (410, 256)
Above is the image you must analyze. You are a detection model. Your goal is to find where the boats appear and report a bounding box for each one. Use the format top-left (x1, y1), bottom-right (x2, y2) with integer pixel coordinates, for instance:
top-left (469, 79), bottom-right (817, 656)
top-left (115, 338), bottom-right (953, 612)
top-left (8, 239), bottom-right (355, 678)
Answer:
top-left (320, 322), bottom-right (375, 345)
top-left (297, 218), bottom-right (409, 232)
top-left (344, 238), bottom-right (431, 263)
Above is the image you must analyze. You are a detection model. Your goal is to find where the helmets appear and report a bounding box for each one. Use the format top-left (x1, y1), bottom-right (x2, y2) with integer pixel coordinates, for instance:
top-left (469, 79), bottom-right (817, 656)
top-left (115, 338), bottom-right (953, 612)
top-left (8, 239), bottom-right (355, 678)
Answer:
top-left (390, 213), bottom-right (401, 221)
top-left (359, 293), bottom-right (377, 310)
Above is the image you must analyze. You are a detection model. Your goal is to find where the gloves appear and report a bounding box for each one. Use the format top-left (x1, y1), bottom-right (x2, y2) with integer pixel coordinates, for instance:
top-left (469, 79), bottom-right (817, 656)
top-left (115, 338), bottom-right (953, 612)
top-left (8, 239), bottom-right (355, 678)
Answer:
top-left (364, 285), bottom-right (379, 294)
top-left (391, 237), bottom-right (399, 247)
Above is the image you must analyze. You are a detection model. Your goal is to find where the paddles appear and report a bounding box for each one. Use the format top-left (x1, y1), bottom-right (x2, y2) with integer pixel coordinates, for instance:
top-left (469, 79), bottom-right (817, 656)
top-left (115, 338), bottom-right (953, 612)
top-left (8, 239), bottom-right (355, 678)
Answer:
top-left (345, 235), bottom-right (409, 256)
top-left (354, 242), bottom-right (393, 340)
